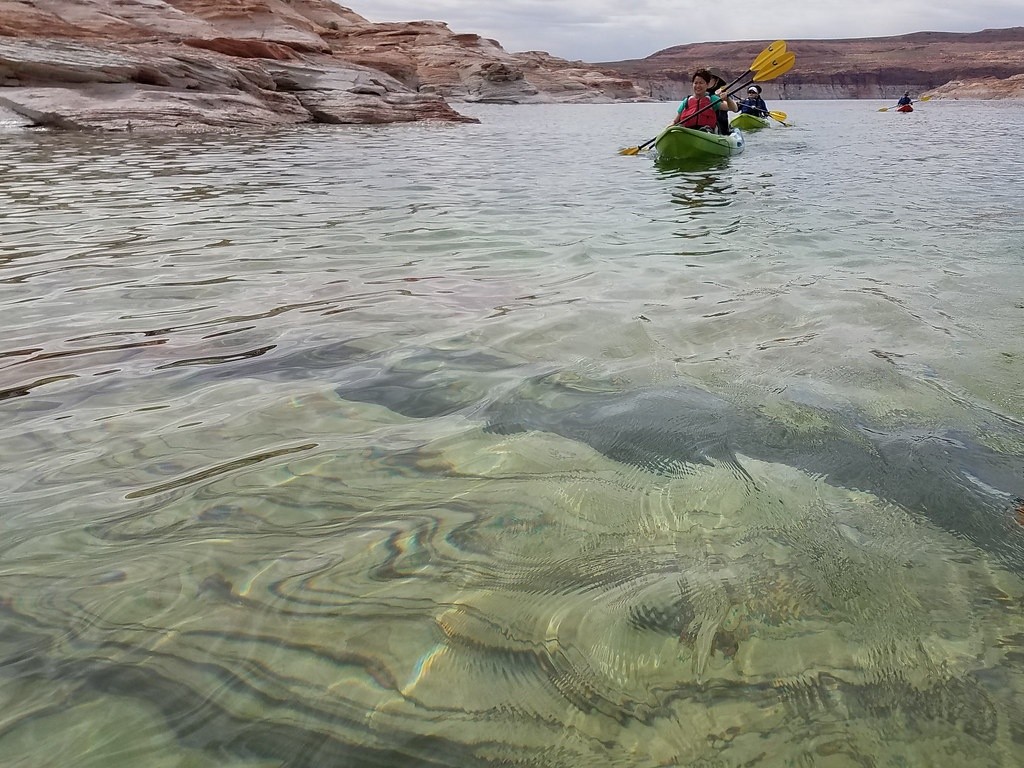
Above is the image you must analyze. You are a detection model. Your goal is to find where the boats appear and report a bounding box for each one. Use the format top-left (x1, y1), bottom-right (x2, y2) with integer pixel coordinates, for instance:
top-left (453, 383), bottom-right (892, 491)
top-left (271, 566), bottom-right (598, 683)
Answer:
top-left (729, 112), bottom-right (771, 130)
top-left (896, 104), bottom-right (913, 112)
top-left (656, 124), bottom-right (744, 160)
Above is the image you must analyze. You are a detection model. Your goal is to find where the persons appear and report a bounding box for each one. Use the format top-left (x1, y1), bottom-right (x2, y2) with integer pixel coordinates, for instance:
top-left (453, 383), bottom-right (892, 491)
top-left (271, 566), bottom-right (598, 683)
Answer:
top-left (736, 85), bottom-right (769, 118)
top-left (897, 91), bottom-right (913, 107)
top-left (672, 67), bottom-right (740, 135)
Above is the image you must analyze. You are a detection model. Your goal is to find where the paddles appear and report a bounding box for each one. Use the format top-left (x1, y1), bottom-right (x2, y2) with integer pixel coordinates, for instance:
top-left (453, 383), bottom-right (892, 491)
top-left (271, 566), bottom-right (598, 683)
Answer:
top-left (722, 40), bottom-right (787, 91)
top-left (737, 103), bottom-right (789, 120)
top-left (620, 37), bottom-right (794, 156)
top-left (879, 94), bottom-right (930, 112)
top-left (732, 95), bottom-right (796, 128)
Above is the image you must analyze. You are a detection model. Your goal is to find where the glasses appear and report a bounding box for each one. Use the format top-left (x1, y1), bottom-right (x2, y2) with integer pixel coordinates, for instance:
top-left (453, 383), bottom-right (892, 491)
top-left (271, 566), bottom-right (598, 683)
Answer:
top-left (748, 91), bottom-right (756, 94)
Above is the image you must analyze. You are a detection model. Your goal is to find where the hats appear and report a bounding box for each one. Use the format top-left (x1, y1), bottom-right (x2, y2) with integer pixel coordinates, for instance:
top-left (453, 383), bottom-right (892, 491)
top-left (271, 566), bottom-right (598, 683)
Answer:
top-left (747, 85), bottom-right (762, 94)
top-left (904, 91), bottom-right (909, 96)
top-left (749, 87), bottom-right (758, 94)
top-left (706, 66), bottom-right (726, 86)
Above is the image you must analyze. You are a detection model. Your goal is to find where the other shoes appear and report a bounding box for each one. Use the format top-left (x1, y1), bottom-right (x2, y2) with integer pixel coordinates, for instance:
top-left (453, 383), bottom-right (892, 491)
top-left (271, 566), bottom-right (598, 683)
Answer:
top-left (700, 127), bottom-right (707, 132)
